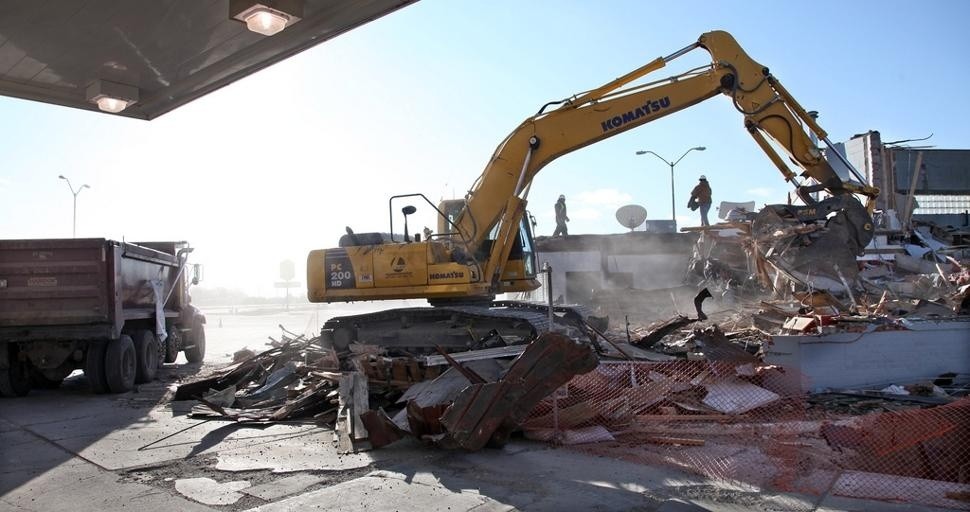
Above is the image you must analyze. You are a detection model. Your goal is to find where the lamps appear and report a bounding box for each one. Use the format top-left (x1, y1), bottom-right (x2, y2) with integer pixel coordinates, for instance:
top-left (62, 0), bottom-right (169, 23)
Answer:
top-left (225, 0), bottom-right (302, 37)
top-left (87, 81), bottom-right (138, 113)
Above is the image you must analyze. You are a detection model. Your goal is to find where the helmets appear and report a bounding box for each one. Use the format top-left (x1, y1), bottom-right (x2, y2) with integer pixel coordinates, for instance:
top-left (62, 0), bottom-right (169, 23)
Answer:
top-left (560, 195), bottom-right (566, 199)
top-left (699, 176), bottom-right (706, 180)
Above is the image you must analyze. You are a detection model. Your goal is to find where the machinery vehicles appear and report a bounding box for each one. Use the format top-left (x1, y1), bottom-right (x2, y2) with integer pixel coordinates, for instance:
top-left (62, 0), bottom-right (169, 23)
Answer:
top-left (307, 30), bottom-right (882, 370)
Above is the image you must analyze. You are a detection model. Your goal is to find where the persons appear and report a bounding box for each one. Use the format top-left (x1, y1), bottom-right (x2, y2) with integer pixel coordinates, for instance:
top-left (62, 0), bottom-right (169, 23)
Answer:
top-left (553, 195), bottom-right (569, 235)
top-left (691, 175), bottom-right (712, 226)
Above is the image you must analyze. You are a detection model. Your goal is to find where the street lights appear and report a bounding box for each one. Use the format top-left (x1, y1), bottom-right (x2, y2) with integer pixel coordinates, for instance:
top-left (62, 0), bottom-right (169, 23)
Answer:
top-left (636, 146), bottom-right (707, 235)
top-left (58, 174), bottom-right (93, 236)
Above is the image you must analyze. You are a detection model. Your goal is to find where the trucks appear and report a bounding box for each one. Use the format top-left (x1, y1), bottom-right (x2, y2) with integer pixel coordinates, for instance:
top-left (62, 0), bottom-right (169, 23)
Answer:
top-left (0, 237), bottom-right (205, 394)
